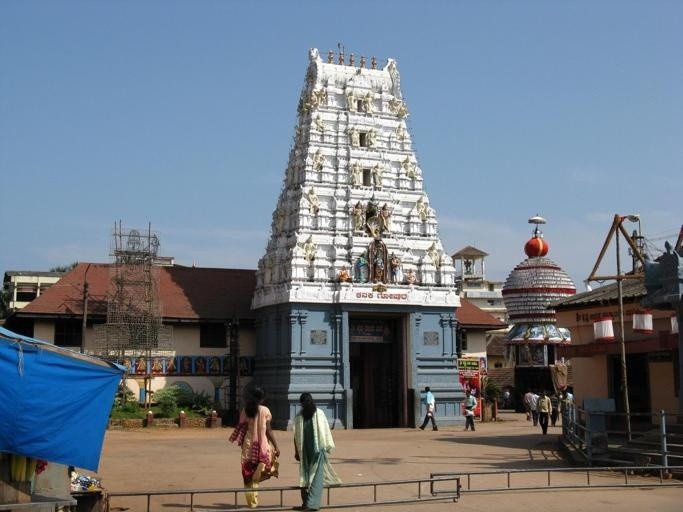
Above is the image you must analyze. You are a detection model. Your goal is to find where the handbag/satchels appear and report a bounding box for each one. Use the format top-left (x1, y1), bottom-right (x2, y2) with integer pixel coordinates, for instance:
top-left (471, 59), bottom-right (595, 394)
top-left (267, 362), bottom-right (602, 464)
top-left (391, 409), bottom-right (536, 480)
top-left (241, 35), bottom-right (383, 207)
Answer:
top-left (252, 451), bottom-right (280, 483)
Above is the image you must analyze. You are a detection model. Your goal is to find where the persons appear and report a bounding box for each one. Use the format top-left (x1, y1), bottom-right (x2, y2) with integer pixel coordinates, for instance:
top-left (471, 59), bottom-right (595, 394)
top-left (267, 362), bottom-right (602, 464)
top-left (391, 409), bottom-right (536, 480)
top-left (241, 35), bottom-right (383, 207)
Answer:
top-left (292, 392), bottom-right (333, 511)
top-left (460, 389), bottom-right (476, 431)
top-left (237, 385), bottom-right (280, 508)
top-left (255, 148), bottom-right (440, 288)
top-left (417, 386), bottom-right (438, 431)
top-left (292, 90), bottom-right (407, 149)
top-left (523, 387), bottom-right (572, 435)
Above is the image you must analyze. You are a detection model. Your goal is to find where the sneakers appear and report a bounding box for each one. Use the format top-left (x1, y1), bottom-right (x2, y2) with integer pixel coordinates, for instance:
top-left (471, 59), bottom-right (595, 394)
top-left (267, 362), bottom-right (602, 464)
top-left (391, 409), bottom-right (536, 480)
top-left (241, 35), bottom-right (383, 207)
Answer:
top-left (533, 423), bottom-right (547, 436)
top-left (463, 428), bottom-right (476, 432)
top-left (551, 424), bottom-right (555, 427)
top-left (419, 427), bottom-right (438, 431)
